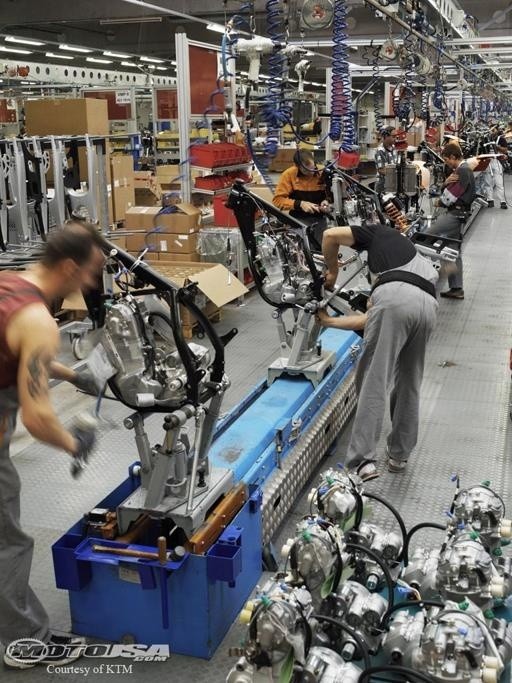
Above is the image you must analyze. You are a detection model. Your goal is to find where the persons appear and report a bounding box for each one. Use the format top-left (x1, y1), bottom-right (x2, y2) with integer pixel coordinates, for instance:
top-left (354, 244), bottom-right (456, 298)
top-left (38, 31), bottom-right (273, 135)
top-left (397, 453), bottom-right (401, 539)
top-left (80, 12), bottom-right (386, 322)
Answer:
top-left (0, 224), bottom-right (104, 669)
top-left (272, 149), bottom-right (332, 255)
top-left (374, 127), bottom-right (396, 192)
top-left (423, 144), bottom-right (476, 300)
top-left (504, 122), bottom-right (512, 174)
top-left (315, 224), bottom-right (438, 483)
top-left (426, 119), bottom-right (455, 147)
top-left (477, 124), bottom-right (508, 209)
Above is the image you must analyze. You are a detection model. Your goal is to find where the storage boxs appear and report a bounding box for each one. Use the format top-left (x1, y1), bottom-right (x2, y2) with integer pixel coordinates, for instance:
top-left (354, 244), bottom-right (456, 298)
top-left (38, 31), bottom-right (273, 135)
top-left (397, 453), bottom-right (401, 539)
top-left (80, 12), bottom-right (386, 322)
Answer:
top-left (126, 202), bottom-right (203, 235)
top-left (22, 98), bottom-right (114, 228)
top-left (124, 232), bottom-right (157, 252)
top-left (61, 260), bottom-right (250, 326)
top-left (157, 252), bottom-right (201, 263)
top-left (154, 233), bottom-right (198, 254)
top-left (126, 250), bottom-right (159, 261)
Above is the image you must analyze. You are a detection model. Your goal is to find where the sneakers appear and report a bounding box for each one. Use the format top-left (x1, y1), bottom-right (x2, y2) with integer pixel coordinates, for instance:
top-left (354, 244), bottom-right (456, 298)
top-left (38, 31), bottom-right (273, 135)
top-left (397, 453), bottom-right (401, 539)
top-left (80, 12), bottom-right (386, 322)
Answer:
top-left (4, 632), bottom-right (87, 669)
top-left (440, 288), bottom-right (465, 300)
top-left (351, 460), bottom-right (380, 481)
top-left (386, 444), bottom-right (407, 473)
